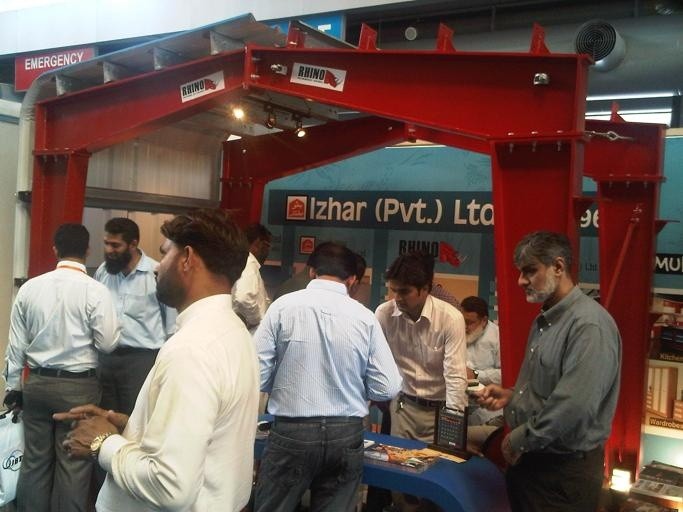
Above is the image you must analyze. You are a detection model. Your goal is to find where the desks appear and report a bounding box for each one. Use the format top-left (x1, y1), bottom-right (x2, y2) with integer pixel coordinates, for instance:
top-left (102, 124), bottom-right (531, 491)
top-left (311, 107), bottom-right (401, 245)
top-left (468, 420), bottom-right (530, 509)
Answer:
top-left (252, 412), bottom-right (511, 510)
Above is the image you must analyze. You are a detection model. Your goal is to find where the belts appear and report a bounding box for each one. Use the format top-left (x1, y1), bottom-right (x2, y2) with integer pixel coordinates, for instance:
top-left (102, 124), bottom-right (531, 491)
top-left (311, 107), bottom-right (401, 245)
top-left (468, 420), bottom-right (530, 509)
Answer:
top-left (521, 444), bottom-right (605, 467)
top-left (400, 391), bottom-right (446, 409)
top-left (28, 366), bottom-right (98, 379)
top-left (274, 415), bottom-right (364, 424)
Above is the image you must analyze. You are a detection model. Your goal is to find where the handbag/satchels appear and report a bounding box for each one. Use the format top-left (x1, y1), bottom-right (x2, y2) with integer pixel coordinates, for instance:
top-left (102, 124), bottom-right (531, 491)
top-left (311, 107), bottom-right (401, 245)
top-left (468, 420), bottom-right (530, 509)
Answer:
top-left (0, 409), bottom-right (26, 507)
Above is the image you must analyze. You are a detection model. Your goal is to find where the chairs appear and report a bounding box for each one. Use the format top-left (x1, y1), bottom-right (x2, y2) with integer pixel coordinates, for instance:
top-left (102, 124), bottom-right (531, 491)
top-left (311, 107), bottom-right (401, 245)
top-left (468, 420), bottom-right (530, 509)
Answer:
top-left (478, 426), bottom-right (505, 474)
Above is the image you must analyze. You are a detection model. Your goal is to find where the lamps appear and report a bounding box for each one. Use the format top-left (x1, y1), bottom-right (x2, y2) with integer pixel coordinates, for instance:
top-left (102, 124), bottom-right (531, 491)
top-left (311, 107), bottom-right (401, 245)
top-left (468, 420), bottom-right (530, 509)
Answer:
top-left (229, 90), bottom-right (306, 139)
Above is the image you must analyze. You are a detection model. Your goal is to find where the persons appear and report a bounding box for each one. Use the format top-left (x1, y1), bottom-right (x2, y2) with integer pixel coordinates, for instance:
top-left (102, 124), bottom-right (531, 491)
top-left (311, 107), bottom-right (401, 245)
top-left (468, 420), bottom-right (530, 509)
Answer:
top-left (252, 242), bottom-right (401, 512)
top-left (371, 248), bottom-right (501, 449)
top-left (474, 230), bottom-right (621, 511)
top-left (5, 217), bottom-right (176, 510)
top-left (52, 211), bottom-right (272, 511)
